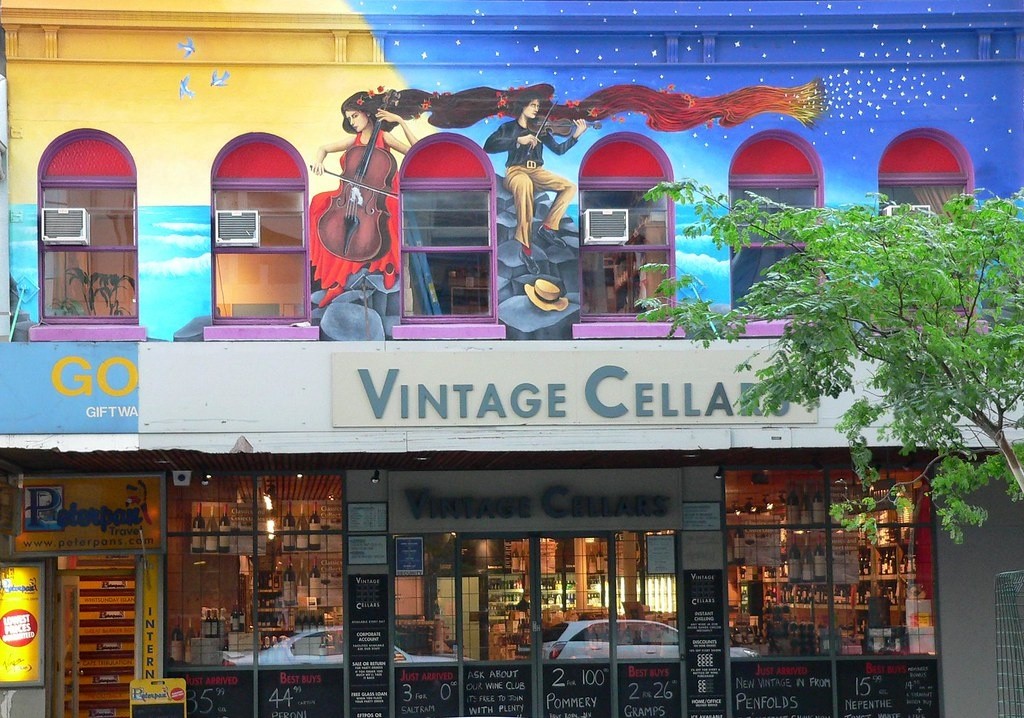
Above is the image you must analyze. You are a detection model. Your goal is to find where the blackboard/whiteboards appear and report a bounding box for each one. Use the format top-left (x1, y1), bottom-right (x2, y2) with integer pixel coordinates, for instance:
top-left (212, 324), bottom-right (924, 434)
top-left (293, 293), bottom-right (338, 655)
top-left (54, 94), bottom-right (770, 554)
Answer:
top-left (731, 660), bottom-right (833, 718)
top-left (542, 664), bottom-right (611, 718)
top-left (616, 662), bottom-right (681, 718)
top-left (394, 667), bottom-right (459, 718)
top-left (835, 660), bottom-right (939, 718)
top-left (465, 665), bottom-right (530, 717)
top-left (259, 669), bottom-right (346, 718)
top-left (164, 668), bottom-right (253, 718)
top-left (132, 703), bottom-right (185, 718)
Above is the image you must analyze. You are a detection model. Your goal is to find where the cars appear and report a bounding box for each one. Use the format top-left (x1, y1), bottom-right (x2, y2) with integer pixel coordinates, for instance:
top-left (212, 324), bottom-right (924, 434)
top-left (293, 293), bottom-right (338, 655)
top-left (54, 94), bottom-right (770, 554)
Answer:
top-left (545, 619), bottom-right (760, 658)
top-left (221, 626), bottom-right (457, 662)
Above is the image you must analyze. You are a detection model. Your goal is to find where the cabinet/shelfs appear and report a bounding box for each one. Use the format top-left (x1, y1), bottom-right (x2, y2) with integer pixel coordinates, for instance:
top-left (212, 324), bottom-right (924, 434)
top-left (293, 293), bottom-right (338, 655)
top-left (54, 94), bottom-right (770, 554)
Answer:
top-left (486, 574), bottom-right (606, 620)
top-left (742, 539), bottom-right (916, 610)
top-left (191, 543), bottom-right (343, 650)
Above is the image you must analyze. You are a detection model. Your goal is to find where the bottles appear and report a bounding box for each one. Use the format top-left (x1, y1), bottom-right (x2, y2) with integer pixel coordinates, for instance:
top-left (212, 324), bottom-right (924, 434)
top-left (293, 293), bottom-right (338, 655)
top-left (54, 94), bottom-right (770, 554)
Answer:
top-left (219, 504), bottom-right (231, 552)
top-left (187, 617), bottom-right (196, 663)
top-left (171, 615), bottom-right (184, 662)
top-left (201, 604), bottom-right (246, 638)
top-left (296, 504), bottom-right (309, 551)
top-left (192, 502), bottom-right (206, 552)
top-left (732, 483), bottom-right (918, 654)
top-left (452, 640), bottom-right (458, 662)
top-left (259, 555), bottom-right (343, 656)
top-left (309, 501), bottom-right (322, 550)
top-left (488, 540), bottom-right (608, 660)
top-left (206, 506), bottom-right (219, 552)
top-left (282, 501), bottom-right (296, 551)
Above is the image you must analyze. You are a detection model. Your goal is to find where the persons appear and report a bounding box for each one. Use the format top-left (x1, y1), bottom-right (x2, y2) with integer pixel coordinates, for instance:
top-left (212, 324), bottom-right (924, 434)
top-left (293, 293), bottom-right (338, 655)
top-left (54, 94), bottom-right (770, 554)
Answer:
top-left (516, 592), bottom-right (531, 611)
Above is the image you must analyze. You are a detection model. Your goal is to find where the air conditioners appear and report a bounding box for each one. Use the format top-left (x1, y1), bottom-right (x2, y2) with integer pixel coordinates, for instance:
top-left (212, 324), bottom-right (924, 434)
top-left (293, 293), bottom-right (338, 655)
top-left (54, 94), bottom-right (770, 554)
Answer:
top-left (214, 210), bottom-right (261, 248)
top-left (41, 208), bottom-right (90, 246)
top-left (582, 209), bottom-right (629, 246)
